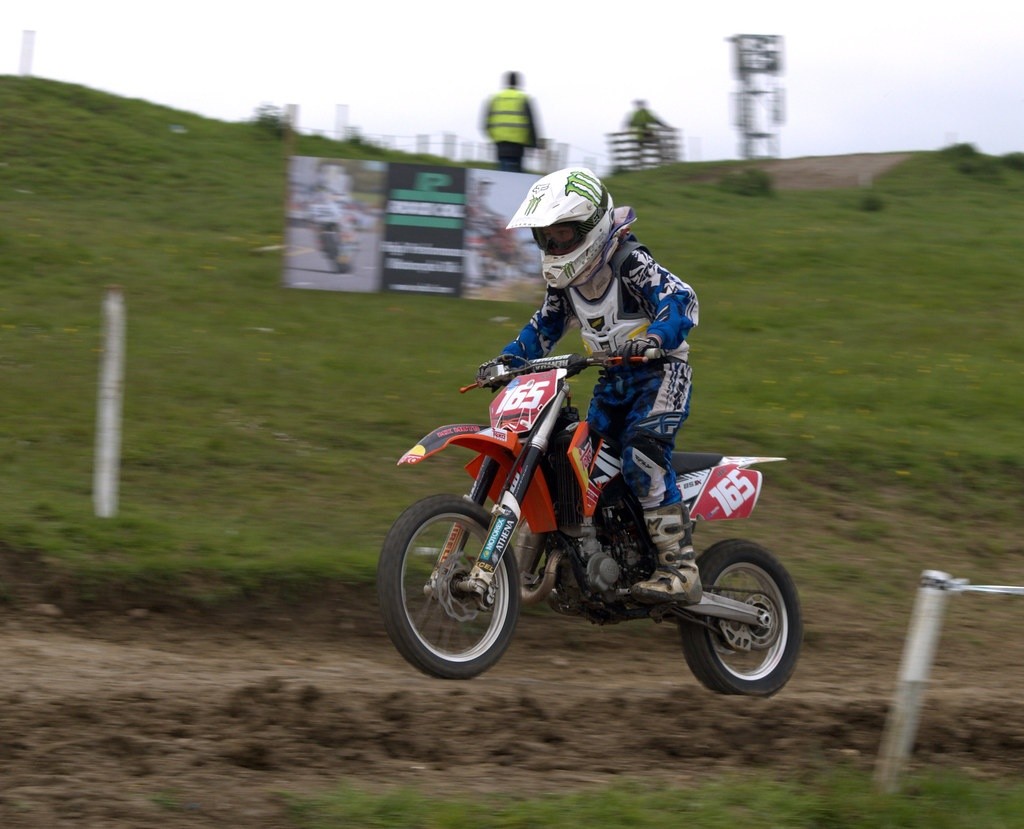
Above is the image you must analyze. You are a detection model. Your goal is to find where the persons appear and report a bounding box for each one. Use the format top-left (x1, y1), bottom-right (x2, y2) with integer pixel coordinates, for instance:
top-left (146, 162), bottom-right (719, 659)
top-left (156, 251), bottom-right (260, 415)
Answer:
top-left (481, 70), bottom-right (537, 170)
top-left (627, 99), bottom-right (667, 160)
top-left (479, 167), bottom-right (703, 603)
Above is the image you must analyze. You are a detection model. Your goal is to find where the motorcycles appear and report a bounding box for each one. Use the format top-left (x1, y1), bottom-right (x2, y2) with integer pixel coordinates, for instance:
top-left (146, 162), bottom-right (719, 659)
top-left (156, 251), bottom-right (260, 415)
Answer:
top-left (376, 349), bottom-right (804, 699)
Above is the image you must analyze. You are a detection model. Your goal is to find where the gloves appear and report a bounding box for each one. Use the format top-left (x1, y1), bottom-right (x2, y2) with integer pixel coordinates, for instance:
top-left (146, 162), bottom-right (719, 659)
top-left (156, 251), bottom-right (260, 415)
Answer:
top-left (476, 355), bottom-right (503, 393)
top-left (609, 337), bottom-right (660, 370)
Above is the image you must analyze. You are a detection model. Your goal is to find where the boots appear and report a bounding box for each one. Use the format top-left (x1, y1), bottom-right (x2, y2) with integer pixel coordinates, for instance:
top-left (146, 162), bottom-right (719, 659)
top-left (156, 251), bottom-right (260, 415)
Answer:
top-left (630, 502), bottom-right (702, 606)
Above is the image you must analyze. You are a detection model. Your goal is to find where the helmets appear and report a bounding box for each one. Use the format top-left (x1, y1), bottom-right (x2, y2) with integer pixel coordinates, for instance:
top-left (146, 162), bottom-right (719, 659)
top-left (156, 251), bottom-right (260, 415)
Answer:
top-left (505, 166), bottom-right (615, 290)
